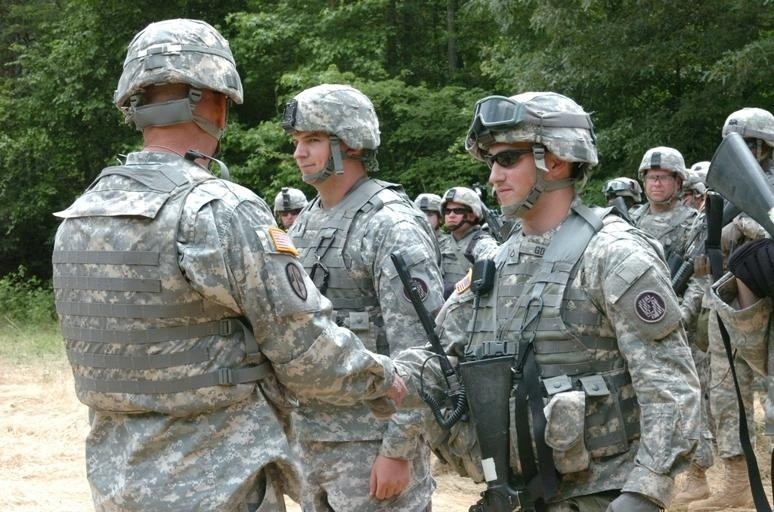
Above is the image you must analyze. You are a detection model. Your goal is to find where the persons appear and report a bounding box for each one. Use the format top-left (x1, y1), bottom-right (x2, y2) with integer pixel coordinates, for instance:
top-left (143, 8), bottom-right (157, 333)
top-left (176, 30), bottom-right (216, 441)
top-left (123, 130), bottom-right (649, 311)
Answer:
top-left (275, 187), bottom-right (309, 230)
top-left (415, 186), bottom-right (498, 476)
top-left (52, 15), bottom-right (422, 511)
top-left (271, 82), bottom-right (448, 510)
top-left (367, 86), bottom-right (708, 511)
top-left (604, 106), bottom-right (774, 512)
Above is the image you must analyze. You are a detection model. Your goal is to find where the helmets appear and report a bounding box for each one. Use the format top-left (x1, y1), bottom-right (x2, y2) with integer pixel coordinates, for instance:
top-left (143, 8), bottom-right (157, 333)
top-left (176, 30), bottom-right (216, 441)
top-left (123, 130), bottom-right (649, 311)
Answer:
top-left (283, 83), bottom-right (381, 150)
top-left (114, 19), bottom-right (243, 112)
top-left (441, 187), bottom-right (484, 221)
top-left (605, 177), bottom-right (642, 203)
top-left (415, 193), bottom-right (442, 217)
top-left (638, 146), bottom-right (687, 181)
top-left (682, 161), bottom-right (712, 191)
top-left (479, 91), bottom-right (598, 169)
top-left (274, 187), bottom-right (309, 215)
top-left (722, 107), bottom-right (774, 146)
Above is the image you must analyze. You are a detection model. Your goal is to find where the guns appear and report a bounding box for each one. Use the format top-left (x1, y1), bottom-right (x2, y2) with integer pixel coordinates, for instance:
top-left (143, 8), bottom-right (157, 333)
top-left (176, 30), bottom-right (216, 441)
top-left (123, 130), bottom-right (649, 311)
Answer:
top-left (705, 132), bottom-right (774, 237)
top-left (607, 195), bottom-right (630, 218)
top-left (479, 201), bottom-right (505, 242)
top-left (389, 248), bottom-right (470, 421)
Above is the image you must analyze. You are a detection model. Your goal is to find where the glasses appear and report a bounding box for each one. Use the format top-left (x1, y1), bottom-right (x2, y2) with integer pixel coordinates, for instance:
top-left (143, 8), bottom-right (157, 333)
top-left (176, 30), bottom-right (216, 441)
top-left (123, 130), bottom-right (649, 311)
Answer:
top-left (643, 174), bottom-right (676, 184)
top-left (483, 148), bottom-right (532, 168)
top-left (444, 207), bottom-right (465, 214)
top-left (279, 210), bottom-right (299, 216)
top-left (466, 95), bottom-right (590, 138)
top-left (603, 180), bottom-right (633, 191)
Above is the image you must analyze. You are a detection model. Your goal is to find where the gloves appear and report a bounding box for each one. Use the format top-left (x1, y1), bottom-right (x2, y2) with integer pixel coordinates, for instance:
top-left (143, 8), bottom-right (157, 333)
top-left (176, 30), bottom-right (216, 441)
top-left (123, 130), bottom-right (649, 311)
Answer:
top-left (685, 253), bottom-right (712, 276)
top-left (607, 494), bottom-right (660, 512)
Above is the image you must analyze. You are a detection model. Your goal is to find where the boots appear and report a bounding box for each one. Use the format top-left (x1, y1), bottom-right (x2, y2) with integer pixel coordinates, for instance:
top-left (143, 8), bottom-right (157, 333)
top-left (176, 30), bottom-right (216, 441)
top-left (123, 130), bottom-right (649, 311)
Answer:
top-left (671, 455), bottom-right (755, 511)
